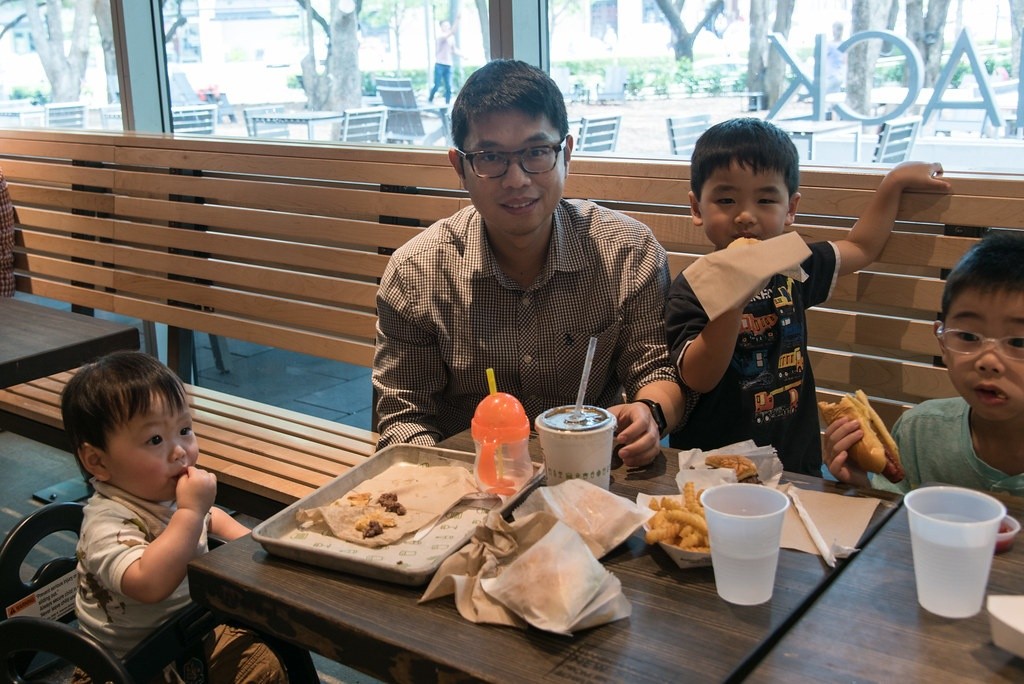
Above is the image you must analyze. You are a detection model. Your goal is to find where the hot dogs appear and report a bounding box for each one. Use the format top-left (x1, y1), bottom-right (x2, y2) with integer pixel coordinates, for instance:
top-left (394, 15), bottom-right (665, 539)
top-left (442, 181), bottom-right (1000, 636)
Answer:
top-left (818, 390), bottom-right (905, 484)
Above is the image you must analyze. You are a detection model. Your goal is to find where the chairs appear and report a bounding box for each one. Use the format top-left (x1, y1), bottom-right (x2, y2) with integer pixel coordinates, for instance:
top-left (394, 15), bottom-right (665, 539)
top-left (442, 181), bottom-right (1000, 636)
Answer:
top-left (0, 502), bottom-right (321, 684)
top-left (46, 76), bottom-right (920, 164)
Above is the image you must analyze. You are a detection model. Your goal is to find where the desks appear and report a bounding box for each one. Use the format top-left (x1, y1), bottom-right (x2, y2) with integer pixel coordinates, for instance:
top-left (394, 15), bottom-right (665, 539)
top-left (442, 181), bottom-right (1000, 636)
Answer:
top-left (188, 424), bottom-right (907, 683)
top-left (0, 297), bottom-right (140, 390)
top-left (740, 480), bottom-right (1024, 684)
top-left (253, 111), bottom-right (344, 142)
top-left (768, 120), bottom-right (865, 161)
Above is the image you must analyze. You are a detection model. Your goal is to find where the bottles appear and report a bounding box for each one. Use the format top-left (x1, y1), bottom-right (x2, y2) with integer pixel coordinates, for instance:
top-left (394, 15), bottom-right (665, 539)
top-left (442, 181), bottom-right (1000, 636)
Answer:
top-left (470, 392), bottom-right (535, 496)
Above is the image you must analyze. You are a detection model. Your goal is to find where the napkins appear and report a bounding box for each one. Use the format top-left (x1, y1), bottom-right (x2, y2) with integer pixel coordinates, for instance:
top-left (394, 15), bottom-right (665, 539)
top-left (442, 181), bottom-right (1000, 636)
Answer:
top-left (769, 482), bottom-right (884, 557)
top-left (418, 501), bottom-right (633, 638)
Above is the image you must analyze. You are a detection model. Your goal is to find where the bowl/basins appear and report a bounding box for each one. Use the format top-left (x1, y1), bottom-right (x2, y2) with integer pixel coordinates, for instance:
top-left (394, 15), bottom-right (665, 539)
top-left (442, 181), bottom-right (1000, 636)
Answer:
top-left (993, 515), bottom-right (1022, 553)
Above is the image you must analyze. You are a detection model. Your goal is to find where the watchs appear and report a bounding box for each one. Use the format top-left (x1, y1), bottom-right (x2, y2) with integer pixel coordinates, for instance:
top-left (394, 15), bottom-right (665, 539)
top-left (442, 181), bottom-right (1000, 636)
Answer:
top-left (633, 399), bottom-right (667, 436)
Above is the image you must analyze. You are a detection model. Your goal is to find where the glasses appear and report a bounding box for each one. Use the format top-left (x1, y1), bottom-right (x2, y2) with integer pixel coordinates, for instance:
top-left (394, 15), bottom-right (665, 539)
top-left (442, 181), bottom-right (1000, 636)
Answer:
top-left (935, 321), bottom-right (1024, 361)
top-left (455, 137), bottom-right (568, 179)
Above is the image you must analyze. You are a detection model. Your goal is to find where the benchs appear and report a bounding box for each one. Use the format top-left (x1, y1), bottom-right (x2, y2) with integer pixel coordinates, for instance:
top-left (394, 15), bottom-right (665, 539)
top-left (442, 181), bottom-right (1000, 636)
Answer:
top-left (1, 129), bottom-right (1023, 519)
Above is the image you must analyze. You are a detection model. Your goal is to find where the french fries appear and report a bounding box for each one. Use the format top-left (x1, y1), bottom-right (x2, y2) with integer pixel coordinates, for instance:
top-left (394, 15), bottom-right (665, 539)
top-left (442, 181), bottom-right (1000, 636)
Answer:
top-left (645, 482), bottom-right (710, 553)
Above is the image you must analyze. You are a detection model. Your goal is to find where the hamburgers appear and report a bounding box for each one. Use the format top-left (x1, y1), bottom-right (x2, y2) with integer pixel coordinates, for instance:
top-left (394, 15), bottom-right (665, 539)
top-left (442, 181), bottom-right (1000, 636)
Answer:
top-left (706, 455), bottom-right (765, 485)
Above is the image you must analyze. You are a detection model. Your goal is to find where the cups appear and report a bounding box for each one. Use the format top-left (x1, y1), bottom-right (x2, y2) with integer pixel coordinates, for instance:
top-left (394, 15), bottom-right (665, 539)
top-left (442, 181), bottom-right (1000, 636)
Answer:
top-left (903, 485), bottom-right (1007, 620)
top-left (699, 482), bottom-right (790, 606)
top-left (532, 404), bottom-right (617, 489)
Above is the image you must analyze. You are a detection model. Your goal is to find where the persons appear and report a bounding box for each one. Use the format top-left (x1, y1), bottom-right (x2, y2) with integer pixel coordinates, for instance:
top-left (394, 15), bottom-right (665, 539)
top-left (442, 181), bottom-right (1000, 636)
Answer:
top-left (667, 118), bottom-right (952, 477)
top-left (59, 352), bottom-right (285, 684)
top-left (826, 22), bottom-right (848, 121)
top-left (428, 13), bottom-right (465, 104)
top-left (0, 170), bottom-right (15, 299)
top-left (371, 60), bottom-right (703, 467)
top-left (823, 232), bottom-right (1024, 518)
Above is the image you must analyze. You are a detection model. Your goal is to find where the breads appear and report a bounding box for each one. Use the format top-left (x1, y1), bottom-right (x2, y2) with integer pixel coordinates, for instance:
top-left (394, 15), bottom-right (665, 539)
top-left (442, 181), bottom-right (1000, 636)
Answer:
top-left (727, 237), bottom-right (762, 249)
